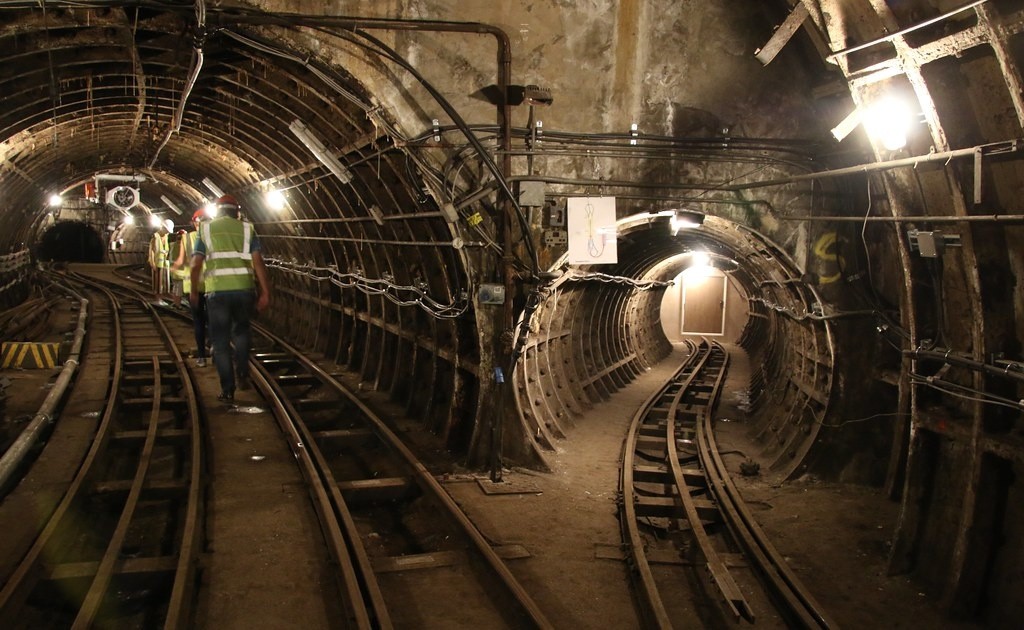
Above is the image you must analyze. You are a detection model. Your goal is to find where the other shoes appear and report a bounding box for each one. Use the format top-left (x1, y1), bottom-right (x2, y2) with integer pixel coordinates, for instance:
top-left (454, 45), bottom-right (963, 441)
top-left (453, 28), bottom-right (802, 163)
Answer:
top-left (238, 375), bottom-right (254, 392)
top-left (216, 382), bottom-right (236, 402)
top-left (153, 299), bottom-right (168, 307)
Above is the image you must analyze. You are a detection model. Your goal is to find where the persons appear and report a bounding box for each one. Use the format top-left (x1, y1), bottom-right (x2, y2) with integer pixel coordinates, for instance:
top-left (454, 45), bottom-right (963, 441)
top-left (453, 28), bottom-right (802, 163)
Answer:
top-left (148, 218), bottom-right (175, 309)
top-left (168, 229), bottom-right (190, 310)
top-left (189, 194), bottom-right (269, 403)
top-left (172, 208), bottom-right (218, 367)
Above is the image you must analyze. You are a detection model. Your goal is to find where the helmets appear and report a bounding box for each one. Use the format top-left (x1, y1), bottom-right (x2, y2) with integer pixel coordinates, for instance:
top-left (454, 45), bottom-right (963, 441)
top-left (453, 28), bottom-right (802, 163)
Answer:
top-left (192, 209), bottom-right (210, 222)
top-left (162, 219), bottom-right (175, 234)
top-left (215, 194), bottom-right (241, 208)
top-left (177, 229), bottom-right (188, 236)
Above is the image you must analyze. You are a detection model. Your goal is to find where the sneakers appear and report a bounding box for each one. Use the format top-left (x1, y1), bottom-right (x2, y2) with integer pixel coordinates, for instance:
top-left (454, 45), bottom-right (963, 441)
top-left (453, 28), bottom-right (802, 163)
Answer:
top-left (175, 306), bottom-right (191, 312)
top-left (169, 303), bottom-right (176, 309)
top-left (208, 343), bottom-right (217, 365)
top-left (196, 358), bottom-right (207, 368)
